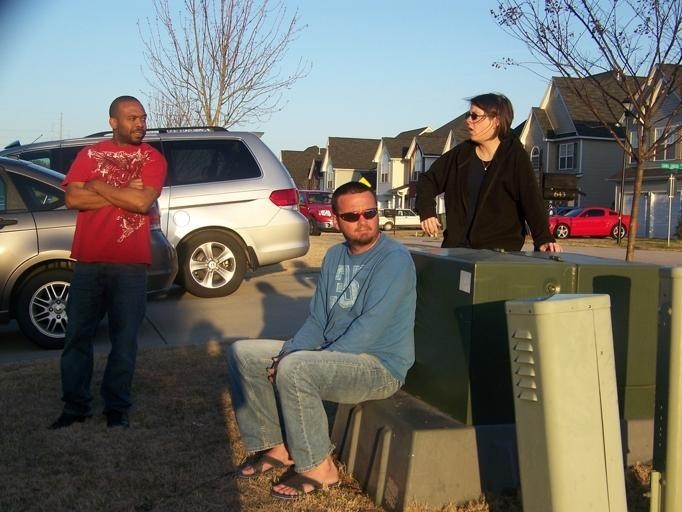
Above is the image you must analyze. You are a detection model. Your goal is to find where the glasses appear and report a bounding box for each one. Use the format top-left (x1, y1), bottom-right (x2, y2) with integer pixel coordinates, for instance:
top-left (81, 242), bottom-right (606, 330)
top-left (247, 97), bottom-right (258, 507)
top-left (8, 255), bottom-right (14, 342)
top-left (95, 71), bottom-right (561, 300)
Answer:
top-left (336, 206), bottom-right (378, 224)
top-left (464, 111), bottom-right (487, 121)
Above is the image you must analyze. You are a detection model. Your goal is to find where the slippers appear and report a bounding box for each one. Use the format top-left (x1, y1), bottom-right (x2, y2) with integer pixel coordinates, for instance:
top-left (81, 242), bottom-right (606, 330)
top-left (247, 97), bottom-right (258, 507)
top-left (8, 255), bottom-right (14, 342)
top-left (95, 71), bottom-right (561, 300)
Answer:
top-left (238, 452), bottom-right (296, 478)
top-left (269, 470), bottom-right (340, 500)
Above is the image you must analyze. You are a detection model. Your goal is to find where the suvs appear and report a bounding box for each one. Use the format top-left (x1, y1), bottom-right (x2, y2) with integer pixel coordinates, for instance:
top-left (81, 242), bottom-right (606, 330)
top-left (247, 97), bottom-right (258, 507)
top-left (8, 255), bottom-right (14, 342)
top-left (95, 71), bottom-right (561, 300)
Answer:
top-left (1, 123), bottom-right (310, 298)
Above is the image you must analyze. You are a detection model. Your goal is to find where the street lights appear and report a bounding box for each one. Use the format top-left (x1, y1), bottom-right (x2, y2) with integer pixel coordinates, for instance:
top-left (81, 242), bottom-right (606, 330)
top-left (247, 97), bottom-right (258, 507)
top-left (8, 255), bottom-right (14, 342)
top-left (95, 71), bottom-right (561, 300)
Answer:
top-left (619, 94), bottom-right (634, 246)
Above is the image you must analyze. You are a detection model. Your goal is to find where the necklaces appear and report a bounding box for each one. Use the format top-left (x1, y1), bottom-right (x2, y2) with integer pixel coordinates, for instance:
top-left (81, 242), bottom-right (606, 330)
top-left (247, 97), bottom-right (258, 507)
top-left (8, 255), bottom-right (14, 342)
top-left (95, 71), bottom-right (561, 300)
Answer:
top-left (478, 148), bottom-right (491, 172)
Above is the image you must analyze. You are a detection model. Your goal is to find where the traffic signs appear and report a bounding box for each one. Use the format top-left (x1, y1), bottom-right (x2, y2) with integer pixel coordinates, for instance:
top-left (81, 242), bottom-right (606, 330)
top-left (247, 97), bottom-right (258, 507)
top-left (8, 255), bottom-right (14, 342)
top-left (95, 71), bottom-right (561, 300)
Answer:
top-left (660, 162), bottom-right (682, 172)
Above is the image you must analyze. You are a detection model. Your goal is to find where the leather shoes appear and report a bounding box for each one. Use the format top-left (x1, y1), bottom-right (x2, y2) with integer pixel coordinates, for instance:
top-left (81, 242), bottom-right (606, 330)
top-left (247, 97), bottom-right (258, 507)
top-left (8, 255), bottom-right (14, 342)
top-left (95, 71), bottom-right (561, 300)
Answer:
top-left (105, 411), bottom-right (131, 429)
top-left (46, 411), bottom-right (94, 431)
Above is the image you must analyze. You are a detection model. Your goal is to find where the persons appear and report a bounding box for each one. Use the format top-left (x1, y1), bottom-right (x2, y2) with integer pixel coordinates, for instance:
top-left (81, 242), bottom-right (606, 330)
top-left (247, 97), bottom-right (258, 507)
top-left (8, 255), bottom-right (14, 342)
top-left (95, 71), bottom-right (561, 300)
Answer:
top-left (416, 93), bottom-right (564, 253)
top-left (225, 180), bottom-right (418, 498)
top-left (48, 96), bottom-right (169, 432)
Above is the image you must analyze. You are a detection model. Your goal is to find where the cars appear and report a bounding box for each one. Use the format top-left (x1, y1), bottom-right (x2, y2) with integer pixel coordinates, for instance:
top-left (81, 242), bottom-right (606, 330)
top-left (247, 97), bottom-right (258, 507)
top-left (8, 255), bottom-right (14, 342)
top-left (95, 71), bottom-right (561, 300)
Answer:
top-left (0, 157), bottom-right (175, 350)
top-left (525, 205), bottom-right (639, 241)
top-left (298, 187), bottom-right (421, 235)
